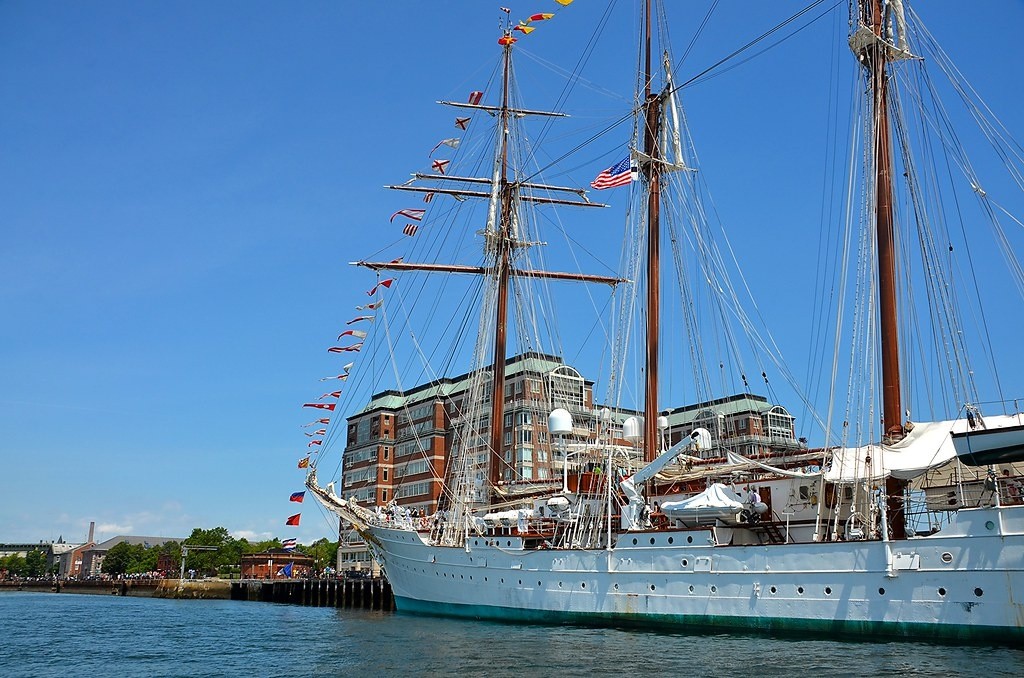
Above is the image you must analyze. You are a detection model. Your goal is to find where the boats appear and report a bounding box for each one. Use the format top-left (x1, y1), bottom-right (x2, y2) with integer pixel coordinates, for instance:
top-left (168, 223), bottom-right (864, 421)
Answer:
top-left (945, 422), bottom-right (1024, 466)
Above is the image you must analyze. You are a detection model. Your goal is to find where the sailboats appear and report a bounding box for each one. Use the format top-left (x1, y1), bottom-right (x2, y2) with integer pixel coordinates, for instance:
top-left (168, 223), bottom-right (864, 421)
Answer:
top-left (302, 1), bottom-right (1023, 644)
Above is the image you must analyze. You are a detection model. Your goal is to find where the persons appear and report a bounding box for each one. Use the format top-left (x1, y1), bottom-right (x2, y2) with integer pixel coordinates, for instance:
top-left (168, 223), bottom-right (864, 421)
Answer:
top-left (0, 564), bottom-right (371, 583)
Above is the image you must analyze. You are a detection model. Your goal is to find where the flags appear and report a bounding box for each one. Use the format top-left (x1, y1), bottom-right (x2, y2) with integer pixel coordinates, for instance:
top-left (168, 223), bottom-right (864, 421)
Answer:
top-left (278, 0), bottom-right (576, 580)
top-left (590, 155), bottom-right (631, 191)
